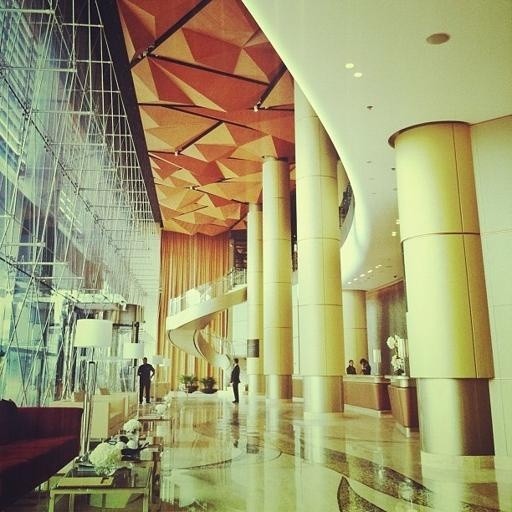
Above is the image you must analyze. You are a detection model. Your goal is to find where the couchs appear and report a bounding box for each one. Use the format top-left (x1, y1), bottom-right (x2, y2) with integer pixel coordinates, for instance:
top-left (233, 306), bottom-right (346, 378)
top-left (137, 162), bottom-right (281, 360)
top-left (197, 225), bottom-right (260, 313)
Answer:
top-left (2, 399), bottom-right (84, 512)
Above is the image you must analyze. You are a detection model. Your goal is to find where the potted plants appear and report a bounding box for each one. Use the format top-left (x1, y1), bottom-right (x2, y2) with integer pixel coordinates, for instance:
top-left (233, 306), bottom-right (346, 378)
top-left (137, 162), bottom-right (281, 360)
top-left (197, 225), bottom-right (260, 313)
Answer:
top-left (200, 376), bottom-right (218, 394)
top-left (176, 374), bottom-right (200, 394)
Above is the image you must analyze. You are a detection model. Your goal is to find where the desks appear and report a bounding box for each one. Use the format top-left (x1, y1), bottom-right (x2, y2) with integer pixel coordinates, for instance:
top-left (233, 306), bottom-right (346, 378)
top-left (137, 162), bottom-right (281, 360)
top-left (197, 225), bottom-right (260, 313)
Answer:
top-left (47, 435), bottom-right (164, 512)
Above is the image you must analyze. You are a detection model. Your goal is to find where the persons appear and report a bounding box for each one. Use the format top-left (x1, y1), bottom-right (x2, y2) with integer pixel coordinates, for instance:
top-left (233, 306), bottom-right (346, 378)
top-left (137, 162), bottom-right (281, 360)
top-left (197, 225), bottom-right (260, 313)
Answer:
top-left (346, 360), bottom-right (356, 375)
top-left (227, 404), bottom-right (240, 448)
top-left (229, 358), bottom-right (241, 404)
top-left (360, 358), bottom-right (371, 375)
top-left (137, 357), bottom-right (155, 404)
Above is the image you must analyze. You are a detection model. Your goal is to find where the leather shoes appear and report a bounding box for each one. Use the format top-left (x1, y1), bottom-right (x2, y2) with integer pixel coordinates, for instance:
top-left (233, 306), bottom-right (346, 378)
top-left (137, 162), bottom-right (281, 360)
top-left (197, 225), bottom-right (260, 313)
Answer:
top-left (232, 400), bottom-right (239, 404)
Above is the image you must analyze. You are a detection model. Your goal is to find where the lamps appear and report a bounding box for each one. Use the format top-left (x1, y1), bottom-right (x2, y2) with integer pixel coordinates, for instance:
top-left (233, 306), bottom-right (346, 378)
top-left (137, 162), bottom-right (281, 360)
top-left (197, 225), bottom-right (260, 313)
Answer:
top-left (151, 355), bottom-right (163, 365)
top-left (64, 319), bottom-right (112, 476)
top-left (124, 342), bottom-right (144, 361)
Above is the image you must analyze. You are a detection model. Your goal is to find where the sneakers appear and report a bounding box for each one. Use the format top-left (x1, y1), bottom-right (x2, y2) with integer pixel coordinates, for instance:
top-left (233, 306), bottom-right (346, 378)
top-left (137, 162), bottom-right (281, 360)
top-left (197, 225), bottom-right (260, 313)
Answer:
top-left (139, 400), bottom-right (151, 404)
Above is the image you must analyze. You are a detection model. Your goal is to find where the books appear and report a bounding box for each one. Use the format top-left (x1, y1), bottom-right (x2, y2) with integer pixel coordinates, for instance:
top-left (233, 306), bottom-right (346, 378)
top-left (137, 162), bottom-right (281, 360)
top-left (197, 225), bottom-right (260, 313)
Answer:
top-left (57, 476), bottom-right (115, 487)
